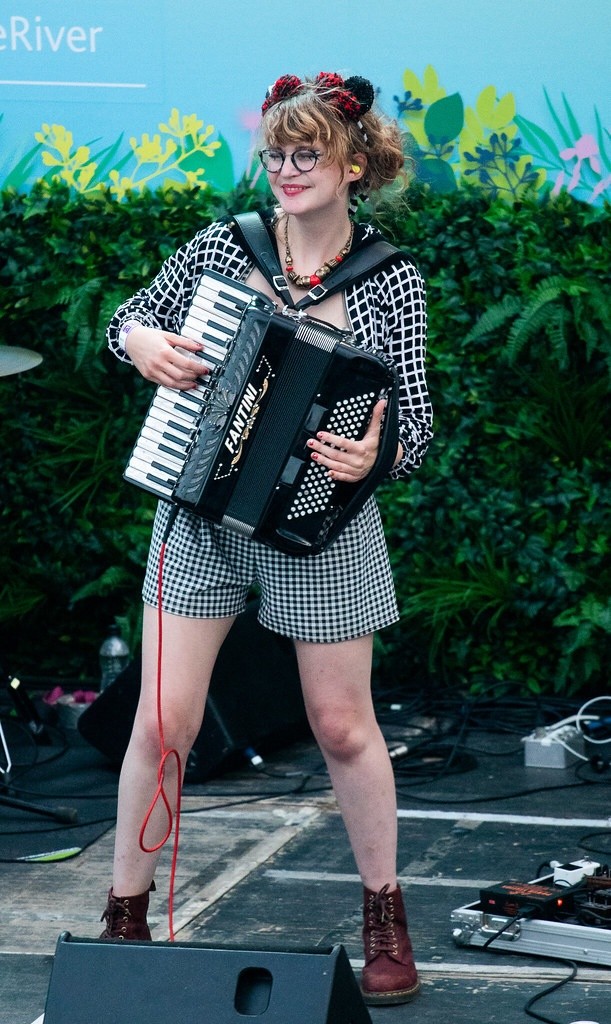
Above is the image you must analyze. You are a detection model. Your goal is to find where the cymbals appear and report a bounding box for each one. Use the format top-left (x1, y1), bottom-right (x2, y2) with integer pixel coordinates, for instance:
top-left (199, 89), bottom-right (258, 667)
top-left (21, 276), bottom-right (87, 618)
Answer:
top-left (0, 344), bottom-right (43, 380)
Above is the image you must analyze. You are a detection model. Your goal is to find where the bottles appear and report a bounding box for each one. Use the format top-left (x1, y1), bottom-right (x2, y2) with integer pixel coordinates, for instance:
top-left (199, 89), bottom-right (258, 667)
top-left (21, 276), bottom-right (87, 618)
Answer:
top-left (98, 626), bottom-right (130, 697)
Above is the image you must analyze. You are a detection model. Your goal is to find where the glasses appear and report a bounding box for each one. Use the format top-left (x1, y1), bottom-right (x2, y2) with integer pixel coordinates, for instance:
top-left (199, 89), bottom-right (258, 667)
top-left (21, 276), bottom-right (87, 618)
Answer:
top-left (259, 147), bottom-right (329, 173)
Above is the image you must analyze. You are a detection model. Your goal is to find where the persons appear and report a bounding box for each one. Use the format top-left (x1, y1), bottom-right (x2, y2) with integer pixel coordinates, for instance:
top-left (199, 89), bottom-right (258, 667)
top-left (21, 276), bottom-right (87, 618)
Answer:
top-left (98, 76), bottom-right (432, 1005)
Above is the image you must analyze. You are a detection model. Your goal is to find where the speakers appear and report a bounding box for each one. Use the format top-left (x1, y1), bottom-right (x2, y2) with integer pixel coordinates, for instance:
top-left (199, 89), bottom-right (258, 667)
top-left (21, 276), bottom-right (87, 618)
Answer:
top-left (77, 652), bottom-right (235, 784)
top-left (39, 931), bottom-right (372, 1024)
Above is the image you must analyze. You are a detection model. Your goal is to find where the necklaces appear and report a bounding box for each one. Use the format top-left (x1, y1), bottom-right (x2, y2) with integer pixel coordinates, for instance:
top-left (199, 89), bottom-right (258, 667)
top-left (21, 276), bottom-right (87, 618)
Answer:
top-left (284, 215), bottom-right (353, 289)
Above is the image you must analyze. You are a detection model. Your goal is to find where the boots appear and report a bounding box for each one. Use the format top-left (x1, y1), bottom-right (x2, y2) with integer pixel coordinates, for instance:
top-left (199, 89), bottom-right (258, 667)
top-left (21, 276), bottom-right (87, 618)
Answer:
top-left (357, 886), bottom-right (420, 1005)
top-left (99, 877), bottom-right (157, 941)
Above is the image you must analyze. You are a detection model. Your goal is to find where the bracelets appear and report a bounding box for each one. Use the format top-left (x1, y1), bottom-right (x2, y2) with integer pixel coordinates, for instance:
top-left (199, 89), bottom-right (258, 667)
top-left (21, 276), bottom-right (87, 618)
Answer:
top-left (119, 320), bottom-right (140, 351)
